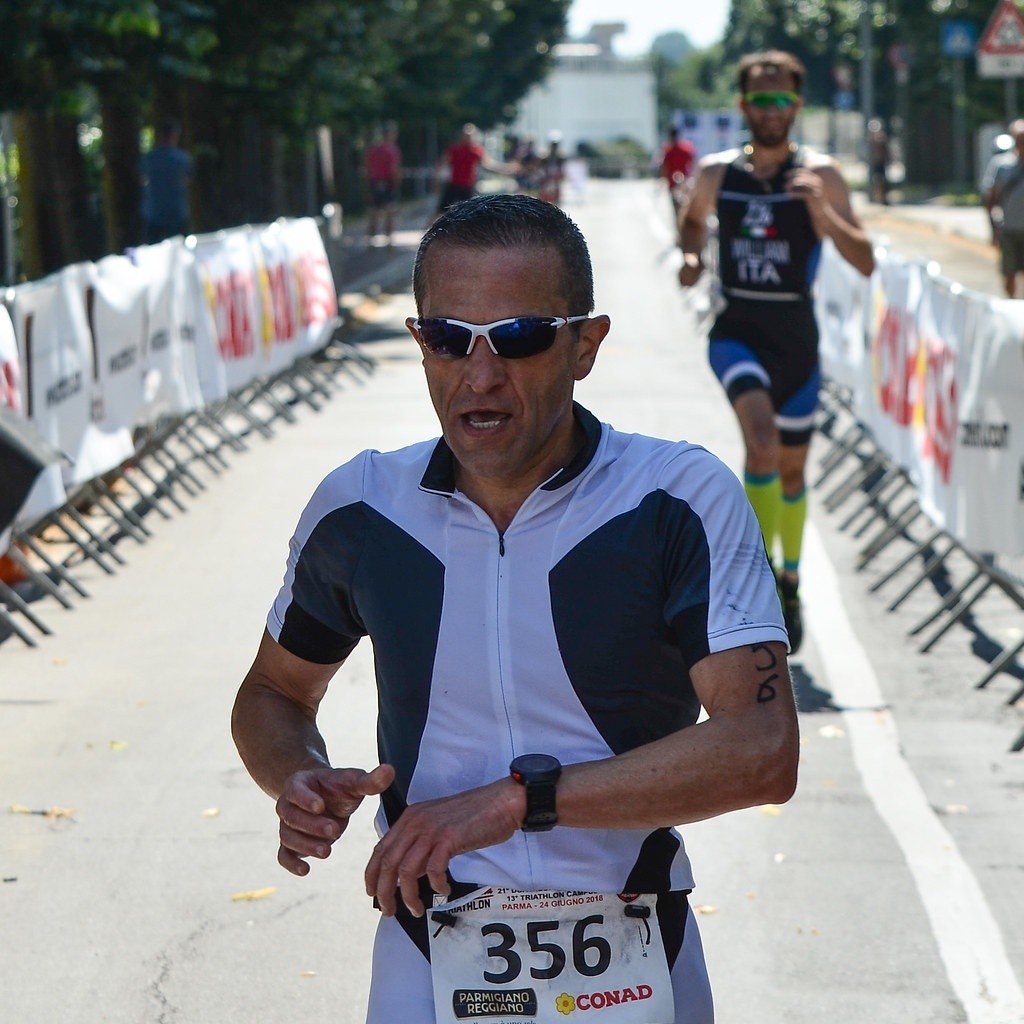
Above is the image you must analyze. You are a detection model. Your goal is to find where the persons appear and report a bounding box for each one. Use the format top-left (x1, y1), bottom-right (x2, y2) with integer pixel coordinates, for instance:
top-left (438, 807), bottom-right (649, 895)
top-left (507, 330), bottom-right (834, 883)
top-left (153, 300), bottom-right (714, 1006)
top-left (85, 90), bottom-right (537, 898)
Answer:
top-left (229, 196), bottom-right (800, 1024)
top-left (867, 118), bottom-right (891, 206)
top-left (676, 51), bottom-right (874, 658)
top-left (983, 119), bottom-right (1024, 300)
top-left (144, 119), bottom-right (195, 243)
top-left (663, 126), bottom-right (694, 249)
top-left (366, 119), bottom-right (401, 251)
top-left (436, 124), bottom-right (587, 210)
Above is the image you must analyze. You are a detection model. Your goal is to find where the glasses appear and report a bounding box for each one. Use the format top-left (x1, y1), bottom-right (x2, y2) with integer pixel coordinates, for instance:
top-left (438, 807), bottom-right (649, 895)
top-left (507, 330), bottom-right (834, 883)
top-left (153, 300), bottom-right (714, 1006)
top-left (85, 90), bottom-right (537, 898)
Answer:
top-left (741, 90), bottom-right (798, 110)
top-left (412, 315), bottom-right (590, 361)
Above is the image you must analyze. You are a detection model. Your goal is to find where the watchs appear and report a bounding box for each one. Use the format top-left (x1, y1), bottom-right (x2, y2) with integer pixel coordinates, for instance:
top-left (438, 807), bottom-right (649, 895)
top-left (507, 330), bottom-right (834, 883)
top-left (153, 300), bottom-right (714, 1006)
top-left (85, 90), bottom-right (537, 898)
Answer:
top-left (509, 753), bottom-right (561, 832)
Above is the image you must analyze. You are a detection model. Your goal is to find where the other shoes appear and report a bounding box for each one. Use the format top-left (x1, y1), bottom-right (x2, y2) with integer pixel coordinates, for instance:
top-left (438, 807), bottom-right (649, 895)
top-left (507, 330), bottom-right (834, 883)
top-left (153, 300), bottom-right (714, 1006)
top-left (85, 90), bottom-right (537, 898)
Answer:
top-left (776, 575), bottom-right (802, 656)
